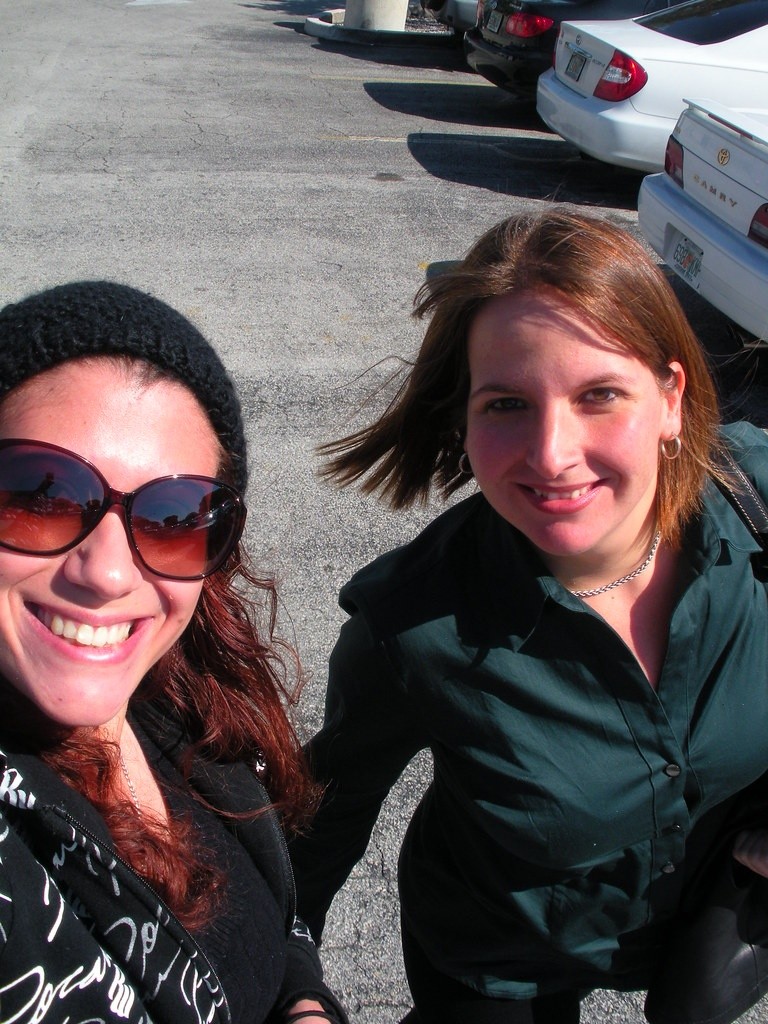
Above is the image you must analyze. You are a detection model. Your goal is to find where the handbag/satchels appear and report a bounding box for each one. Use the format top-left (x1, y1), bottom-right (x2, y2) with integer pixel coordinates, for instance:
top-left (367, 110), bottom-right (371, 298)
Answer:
top-left (643, 864), bottom-right (767, 1024)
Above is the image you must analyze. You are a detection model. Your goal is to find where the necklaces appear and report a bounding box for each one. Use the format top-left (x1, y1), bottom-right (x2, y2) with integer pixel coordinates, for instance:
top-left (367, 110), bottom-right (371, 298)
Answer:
top-left (569, 524), bottom-right (663, 597)
top-left (117, 747), bottom-right (142, 816)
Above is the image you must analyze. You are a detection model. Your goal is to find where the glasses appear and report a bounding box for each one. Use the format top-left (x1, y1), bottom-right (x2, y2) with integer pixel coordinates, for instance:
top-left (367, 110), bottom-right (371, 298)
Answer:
top-left (0, 438), bottom-right (246, 583)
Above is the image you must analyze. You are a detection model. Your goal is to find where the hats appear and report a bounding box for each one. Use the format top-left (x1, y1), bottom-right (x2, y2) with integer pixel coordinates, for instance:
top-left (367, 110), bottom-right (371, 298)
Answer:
top-left (1, 280), bottom-right (250, 457)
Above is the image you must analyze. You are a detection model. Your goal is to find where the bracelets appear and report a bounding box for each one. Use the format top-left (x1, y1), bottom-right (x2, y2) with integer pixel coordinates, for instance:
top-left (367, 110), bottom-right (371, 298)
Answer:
top-left (287, 1010), bottom-right (339, 1024)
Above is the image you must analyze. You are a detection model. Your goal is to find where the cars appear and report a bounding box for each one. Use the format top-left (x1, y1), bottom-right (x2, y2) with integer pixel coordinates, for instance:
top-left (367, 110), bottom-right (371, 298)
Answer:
top-left (534, 1), bottom-right (768, 174)
top-left (461, 0), bottom-right (686, 99)
top-left (636, 98), bottom-right (768, 358)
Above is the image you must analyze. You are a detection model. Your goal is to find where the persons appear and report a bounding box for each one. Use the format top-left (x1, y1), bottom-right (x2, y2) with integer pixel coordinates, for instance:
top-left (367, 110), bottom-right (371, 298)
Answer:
top-left (261, 211), bottom-right (768, 1024)
top-left (0, 278), bottom-right (352, 1024)
